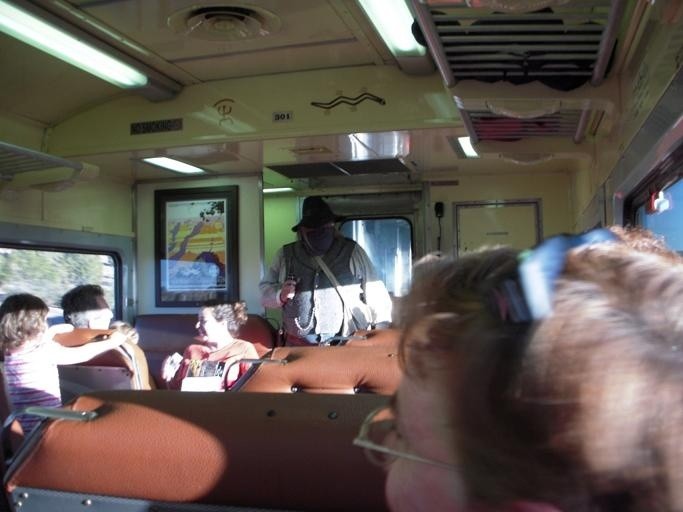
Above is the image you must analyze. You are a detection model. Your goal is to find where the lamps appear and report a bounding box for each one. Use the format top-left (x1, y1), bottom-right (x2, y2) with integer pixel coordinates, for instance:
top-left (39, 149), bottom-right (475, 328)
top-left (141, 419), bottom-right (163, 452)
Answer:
top-left (1, 0), bottom-right (184, 108)
top-left (355, 0), bottom-right (438, 75)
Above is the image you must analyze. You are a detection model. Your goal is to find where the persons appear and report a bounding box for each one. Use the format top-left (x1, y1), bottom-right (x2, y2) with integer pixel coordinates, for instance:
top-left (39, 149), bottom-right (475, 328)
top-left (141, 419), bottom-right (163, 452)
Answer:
top-left (59, 283), bottom-right (145, 394)
top-left (156, 299), bottom-right (261, 393)
top-left (349, 221), bottom-right (683, 511)
top-left (255, 193), bottom-right (395, 347)
top-left (0, 293), bottom-right (139, 442)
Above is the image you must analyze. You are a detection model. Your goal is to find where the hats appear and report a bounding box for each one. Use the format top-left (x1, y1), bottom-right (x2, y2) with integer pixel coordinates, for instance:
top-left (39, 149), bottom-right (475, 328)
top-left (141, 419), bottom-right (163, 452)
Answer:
top-left (292, 197), bottom-right (346, 231)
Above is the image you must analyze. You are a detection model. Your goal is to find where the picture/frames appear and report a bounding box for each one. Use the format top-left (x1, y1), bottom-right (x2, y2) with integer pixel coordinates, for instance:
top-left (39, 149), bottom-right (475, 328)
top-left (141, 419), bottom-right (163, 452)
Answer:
top-left (152, 186), bottom-right (242, 308)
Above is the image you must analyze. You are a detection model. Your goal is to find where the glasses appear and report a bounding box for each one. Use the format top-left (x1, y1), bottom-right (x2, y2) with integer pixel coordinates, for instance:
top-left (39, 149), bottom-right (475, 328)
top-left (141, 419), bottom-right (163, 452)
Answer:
top-left (354, 405), bottom-right (464, 473)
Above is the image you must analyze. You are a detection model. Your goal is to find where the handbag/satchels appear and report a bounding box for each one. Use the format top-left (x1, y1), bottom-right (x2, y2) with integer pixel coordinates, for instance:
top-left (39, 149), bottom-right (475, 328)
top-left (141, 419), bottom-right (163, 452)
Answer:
top-left (342, 299), bottom-right (372, 343)
